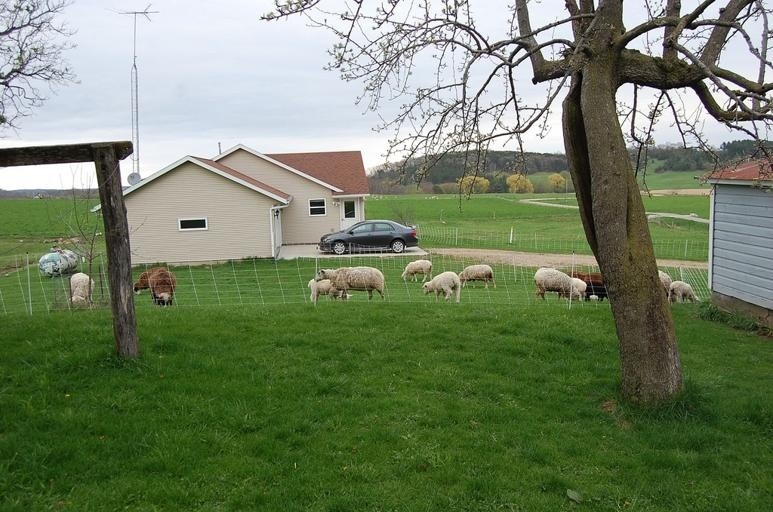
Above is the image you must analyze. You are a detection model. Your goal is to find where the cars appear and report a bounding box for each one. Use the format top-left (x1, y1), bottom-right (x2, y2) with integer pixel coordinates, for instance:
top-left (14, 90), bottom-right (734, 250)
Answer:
top-left (316, 218), bottom-right (420, 253)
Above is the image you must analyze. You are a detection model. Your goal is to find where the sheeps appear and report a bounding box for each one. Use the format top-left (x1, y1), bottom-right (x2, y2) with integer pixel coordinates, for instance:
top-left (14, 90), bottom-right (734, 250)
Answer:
top-left (307, 266), bottom-right (387, 303)
top-left (658, 269), bottom-right (675, 304)
top-left (148, 267), bottom-right (176, 308)
top-left (400, 259), bottom-right (433, 285)
top-left (66, 272), bottom-right (95, 309)
top-left (457, 265), bottom-right (497, 290)
top-left (533, 266), bottom-right (608, 302)
top-left (132, 267), bottom-right (160, 295)
top-left (420, 271), bottom-right (463, 304)
top-left (667, 280), bottom-right (701, 304)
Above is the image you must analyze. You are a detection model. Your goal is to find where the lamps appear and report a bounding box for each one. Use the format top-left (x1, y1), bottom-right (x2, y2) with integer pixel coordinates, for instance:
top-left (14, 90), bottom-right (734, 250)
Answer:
top-left (274, 209), bottom-right (279, 219)
